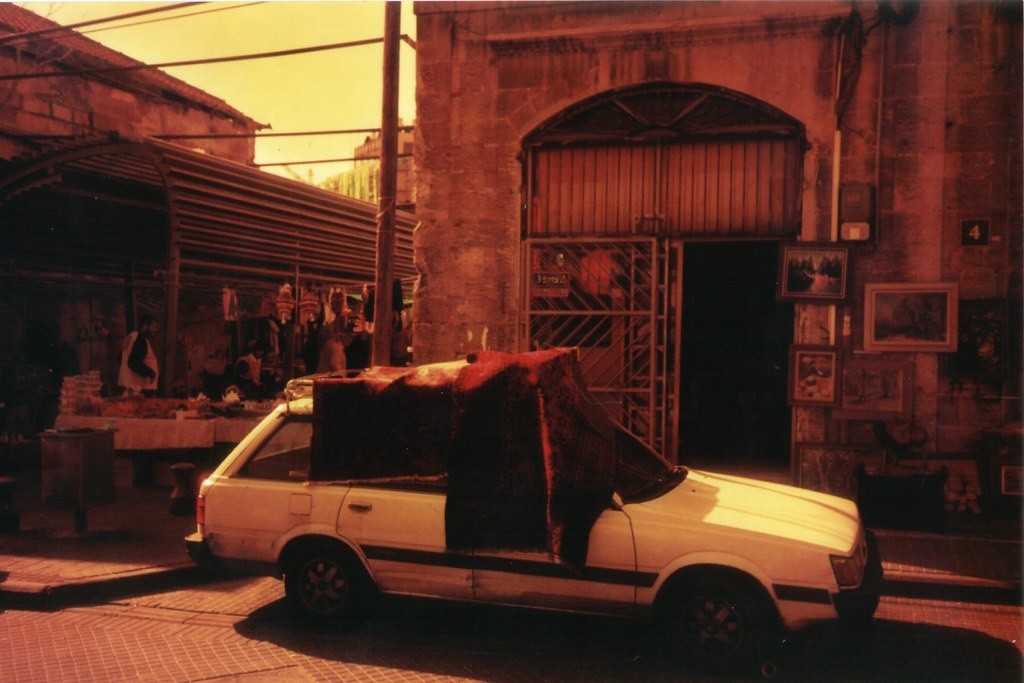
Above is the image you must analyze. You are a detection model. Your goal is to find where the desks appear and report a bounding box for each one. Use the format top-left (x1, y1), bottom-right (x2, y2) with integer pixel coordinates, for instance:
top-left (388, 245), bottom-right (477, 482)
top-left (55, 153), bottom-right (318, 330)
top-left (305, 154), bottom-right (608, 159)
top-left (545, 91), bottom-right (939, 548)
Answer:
top-left (53, 413), bottom-right (260, 448)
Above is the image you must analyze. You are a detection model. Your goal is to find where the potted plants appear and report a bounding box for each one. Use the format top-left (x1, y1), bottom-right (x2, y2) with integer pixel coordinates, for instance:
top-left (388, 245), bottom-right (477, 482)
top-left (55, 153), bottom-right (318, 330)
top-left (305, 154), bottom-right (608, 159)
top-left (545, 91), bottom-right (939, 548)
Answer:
top-left (37, 425), bottom-right (119, 505)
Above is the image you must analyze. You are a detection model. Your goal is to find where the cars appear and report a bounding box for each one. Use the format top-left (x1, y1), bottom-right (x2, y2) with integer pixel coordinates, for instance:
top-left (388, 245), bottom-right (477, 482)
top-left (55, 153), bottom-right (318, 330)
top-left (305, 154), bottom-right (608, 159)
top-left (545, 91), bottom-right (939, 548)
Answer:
top-left (183, 347), bottom-right (888, 674)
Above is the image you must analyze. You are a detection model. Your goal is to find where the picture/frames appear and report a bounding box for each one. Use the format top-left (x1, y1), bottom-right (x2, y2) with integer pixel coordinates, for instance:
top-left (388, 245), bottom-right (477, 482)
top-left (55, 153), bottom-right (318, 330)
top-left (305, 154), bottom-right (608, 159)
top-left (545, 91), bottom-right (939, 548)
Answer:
top-left (864, 283), bottom-right (960, 353)
top-left (832, 360), bottom-right (916, 420)
top-left (776, 240), bottom-right (853, 304)
top-left (790, 299), bottom-right (843, 350)
top-left (795, 441), bottom-right (889, 528)
top-left (1000, 465), bottom-right (1024, 496)
top-left (788, 344), bottom-right (843, 409)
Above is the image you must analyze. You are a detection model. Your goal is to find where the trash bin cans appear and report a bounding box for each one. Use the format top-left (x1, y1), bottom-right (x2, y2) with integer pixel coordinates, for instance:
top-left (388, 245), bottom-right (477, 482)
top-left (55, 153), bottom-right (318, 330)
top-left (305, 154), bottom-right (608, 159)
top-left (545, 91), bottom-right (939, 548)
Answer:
top-left (38, 426), bottom-right (120, 510)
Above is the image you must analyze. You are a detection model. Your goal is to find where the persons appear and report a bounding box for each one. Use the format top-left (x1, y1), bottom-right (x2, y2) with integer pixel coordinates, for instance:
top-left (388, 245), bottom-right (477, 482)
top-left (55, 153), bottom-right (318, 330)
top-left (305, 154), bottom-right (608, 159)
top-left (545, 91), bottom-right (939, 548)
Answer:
top-left (233, 334), bottom-right (272, 391)
top-left (117, 312), bottom-right (165, 490)
top-left (316, 323), bottom-right (359, 374)
